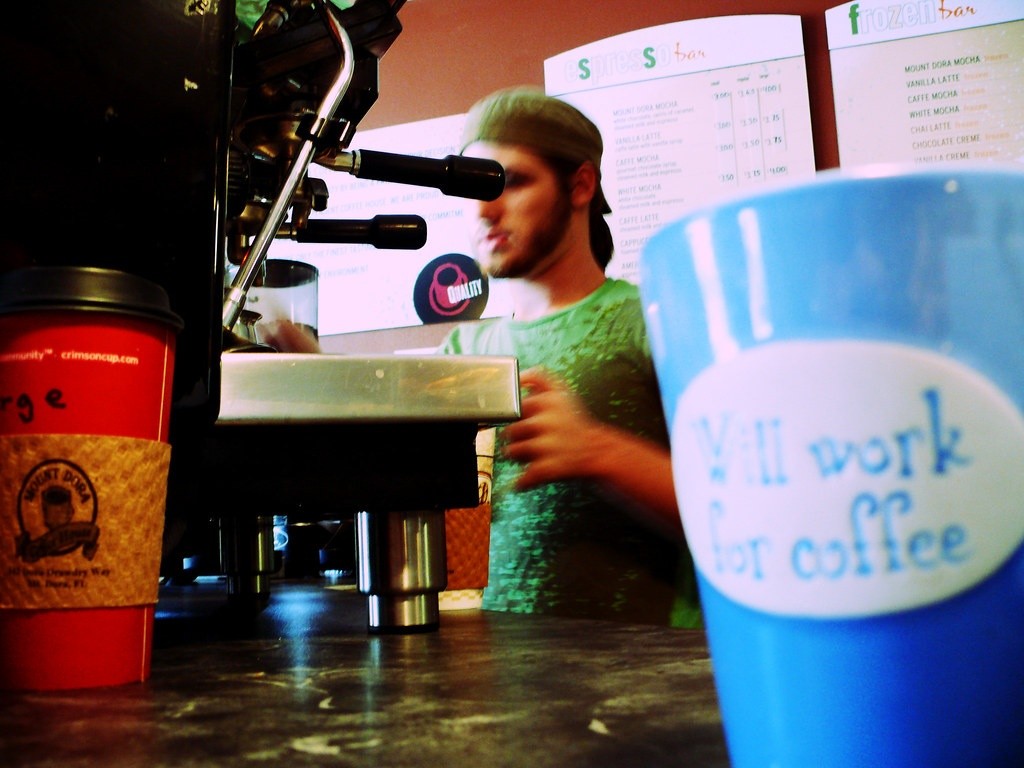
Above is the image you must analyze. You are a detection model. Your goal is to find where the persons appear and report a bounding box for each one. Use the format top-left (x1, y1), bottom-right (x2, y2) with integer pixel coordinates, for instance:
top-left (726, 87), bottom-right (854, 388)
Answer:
top-left (437, 95), bottom-right (683, 626)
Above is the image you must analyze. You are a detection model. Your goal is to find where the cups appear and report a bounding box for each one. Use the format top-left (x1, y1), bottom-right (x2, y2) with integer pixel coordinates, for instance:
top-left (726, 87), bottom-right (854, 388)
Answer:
top-left (637, 158), bottom-right (1024, 768)
top-left (224, 259), bottom-right (319, 356)
top-left (0, 264), bottom-right (184, 693)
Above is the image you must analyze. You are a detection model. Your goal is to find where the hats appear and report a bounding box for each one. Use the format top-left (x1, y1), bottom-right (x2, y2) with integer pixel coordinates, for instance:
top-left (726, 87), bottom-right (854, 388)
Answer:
top-left (456, 85), bottom-right (614, 215)
